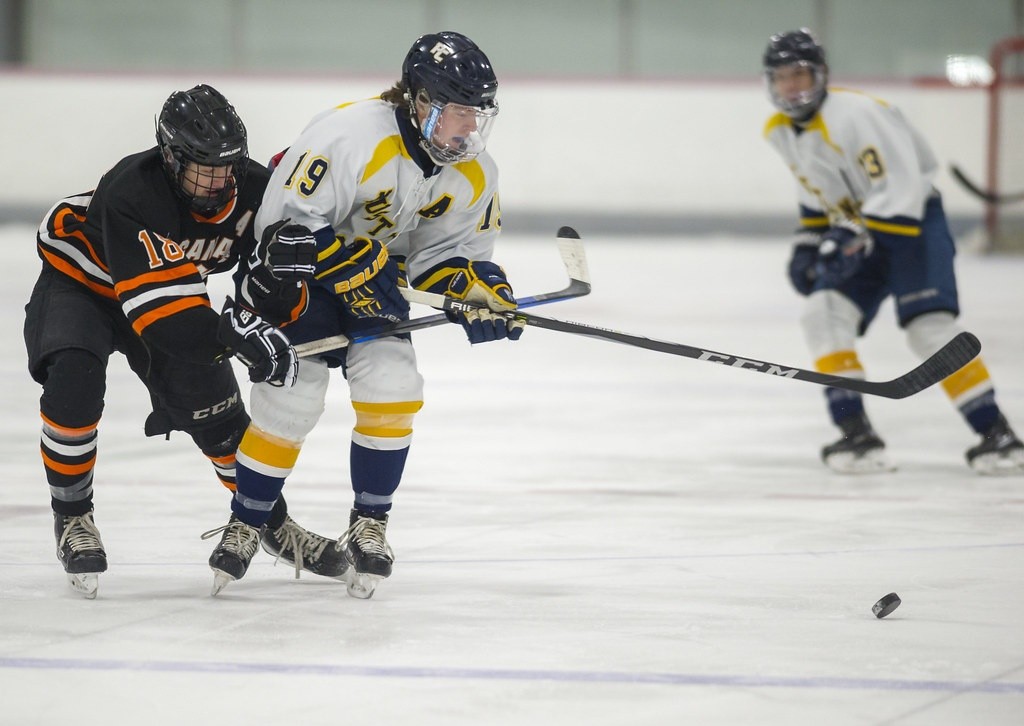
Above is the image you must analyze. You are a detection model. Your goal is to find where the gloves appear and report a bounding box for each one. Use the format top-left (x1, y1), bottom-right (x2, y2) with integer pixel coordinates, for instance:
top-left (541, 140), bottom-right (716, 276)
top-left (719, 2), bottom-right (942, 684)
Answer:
top-left (815, 227), bottom-right (867, 283)
top-left (315, 235), bottom-right (409, 323)
top-left (444, 261), bottom-right (526, 344)
top-left (788, 244), bottom-right (823, 295)
top-left (247, 224), bottom-right (316, 294)
top-left (217, 295), bottom-right (298, 387)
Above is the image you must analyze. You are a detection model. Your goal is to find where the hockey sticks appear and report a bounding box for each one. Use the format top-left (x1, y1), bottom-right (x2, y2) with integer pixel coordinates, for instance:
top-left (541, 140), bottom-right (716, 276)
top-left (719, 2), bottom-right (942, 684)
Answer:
top-left (397, 284), bottom-right (983, 400)
top-left (290, 225), bottom-right (593, 358)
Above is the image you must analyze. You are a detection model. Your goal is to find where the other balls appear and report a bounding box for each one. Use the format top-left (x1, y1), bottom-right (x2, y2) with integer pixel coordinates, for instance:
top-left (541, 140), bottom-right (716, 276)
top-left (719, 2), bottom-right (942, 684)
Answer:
top-left (871, 592), bottom-right (901, 619)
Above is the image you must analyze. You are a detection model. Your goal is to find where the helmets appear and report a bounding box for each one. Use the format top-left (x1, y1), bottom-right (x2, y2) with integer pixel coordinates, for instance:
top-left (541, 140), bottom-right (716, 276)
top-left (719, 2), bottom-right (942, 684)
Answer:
top-left (763, 26), bottom-right (826, 120)
top-left (402, 31), bottom-right (499, 167)
top-left (154, 82), bottom-right (250, 219)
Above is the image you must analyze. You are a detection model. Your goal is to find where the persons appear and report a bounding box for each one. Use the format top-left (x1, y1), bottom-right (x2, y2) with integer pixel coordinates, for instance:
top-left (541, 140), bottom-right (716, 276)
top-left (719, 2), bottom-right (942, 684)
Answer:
top-left (23, 84), bottom-right (351, 600)
top-left (760, 29), bottom-right (1023, 474)
top-left (209, 31), bottom-right (523, 598)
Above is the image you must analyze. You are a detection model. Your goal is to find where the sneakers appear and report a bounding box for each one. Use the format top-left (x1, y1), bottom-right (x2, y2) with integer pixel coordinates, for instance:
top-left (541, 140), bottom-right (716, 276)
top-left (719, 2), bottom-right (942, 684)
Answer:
top-left (823, 425), bottom-right (887, 474)
top-left (200, 511), bottom-right (268, 597)
top-left (259, 513), bottom-right (356, 582)
top-left (967, 424), bottom-right (1024, 475)
top-left (334, 508), bottom-right (396, 599)
top-left (54, 504), bottom-right (108, 599)
